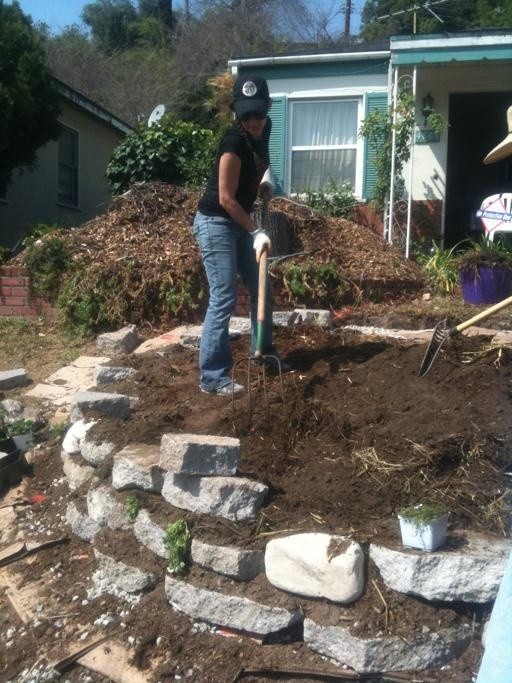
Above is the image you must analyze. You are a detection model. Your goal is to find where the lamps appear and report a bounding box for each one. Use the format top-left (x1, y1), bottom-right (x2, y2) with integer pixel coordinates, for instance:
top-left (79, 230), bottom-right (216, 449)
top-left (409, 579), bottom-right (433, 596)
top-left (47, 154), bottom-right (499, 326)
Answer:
top-left (480, 191), bottom-right (512, 251)
top-left (419, 89), bottom-right (435, 125)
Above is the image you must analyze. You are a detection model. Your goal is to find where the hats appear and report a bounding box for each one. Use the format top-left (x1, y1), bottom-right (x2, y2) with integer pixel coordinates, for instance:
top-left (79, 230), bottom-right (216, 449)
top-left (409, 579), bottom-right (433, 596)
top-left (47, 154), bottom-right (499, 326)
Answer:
top-left (233, 75), bottom-right (268, 112)
top-left (483, 105), bottom-right (512, 165)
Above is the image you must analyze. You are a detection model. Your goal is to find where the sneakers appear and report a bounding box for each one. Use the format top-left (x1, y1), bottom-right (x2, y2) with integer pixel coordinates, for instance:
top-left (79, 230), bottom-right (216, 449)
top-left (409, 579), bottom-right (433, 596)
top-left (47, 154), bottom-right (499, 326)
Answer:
top-left (200, 382), bottom-right (244, 395)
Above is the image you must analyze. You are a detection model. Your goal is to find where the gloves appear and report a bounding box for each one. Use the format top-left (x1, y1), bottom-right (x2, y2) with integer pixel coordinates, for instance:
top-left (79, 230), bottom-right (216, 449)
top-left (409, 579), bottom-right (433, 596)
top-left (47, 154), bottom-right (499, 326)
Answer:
top-left (249, 229), bottom-right (272, 263)
top-left (260, 168), bottom-right (273, 200)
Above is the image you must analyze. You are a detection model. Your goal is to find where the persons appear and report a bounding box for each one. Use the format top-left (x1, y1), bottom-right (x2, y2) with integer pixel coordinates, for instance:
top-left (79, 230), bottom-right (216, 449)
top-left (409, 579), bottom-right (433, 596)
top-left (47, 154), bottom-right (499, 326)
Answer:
top-left (192, 73), bottom-right (291, 400)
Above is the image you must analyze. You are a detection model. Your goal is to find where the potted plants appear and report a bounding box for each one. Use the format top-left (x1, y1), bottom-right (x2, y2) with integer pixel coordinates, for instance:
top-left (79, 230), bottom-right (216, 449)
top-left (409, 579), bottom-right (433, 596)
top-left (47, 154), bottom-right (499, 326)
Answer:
top-left (1, 400), bottom-right (38, 458)
top-left (472, 233), bottom-right (512, 303)
top-left (452, 247), bottom-right (480, 305)
top-left (397, 502), bottom-right (453, 551)
